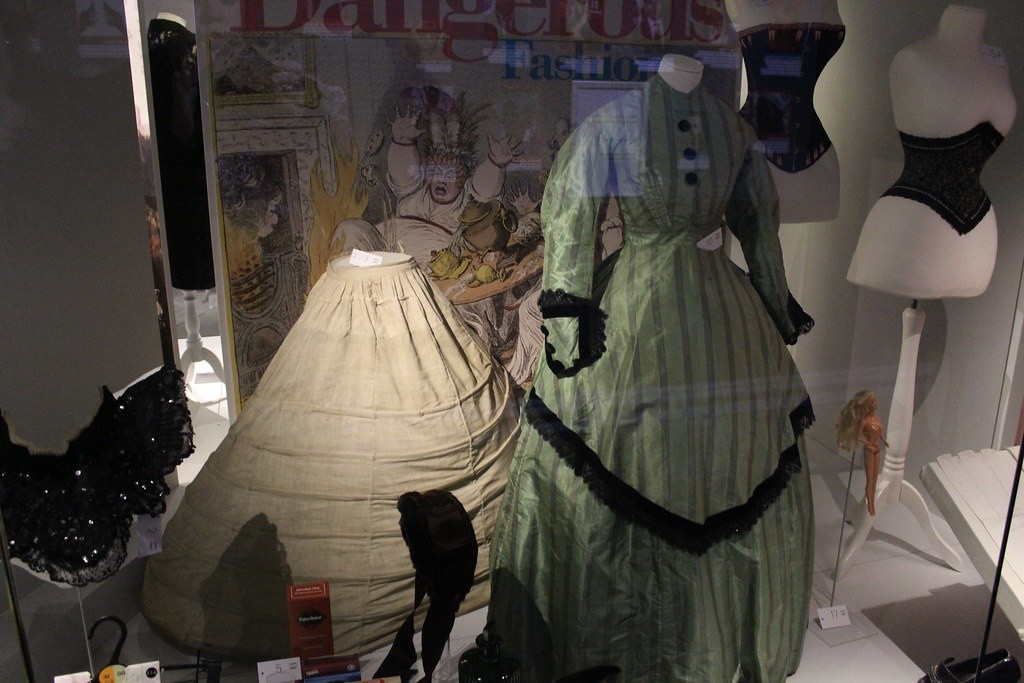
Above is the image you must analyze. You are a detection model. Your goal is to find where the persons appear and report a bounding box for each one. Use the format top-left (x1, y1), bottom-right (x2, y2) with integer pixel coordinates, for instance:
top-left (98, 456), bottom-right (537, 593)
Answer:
top-left (725, 0), bottom-right (843, 224)
top-left (483, 51), bottom-right (823, 683)
top-left (143, 11), bottom-right (228, 395)
top-left (837, 388), bottom-right (890, 517)
top-left (848, 3), bottom-right (1019, 300)
